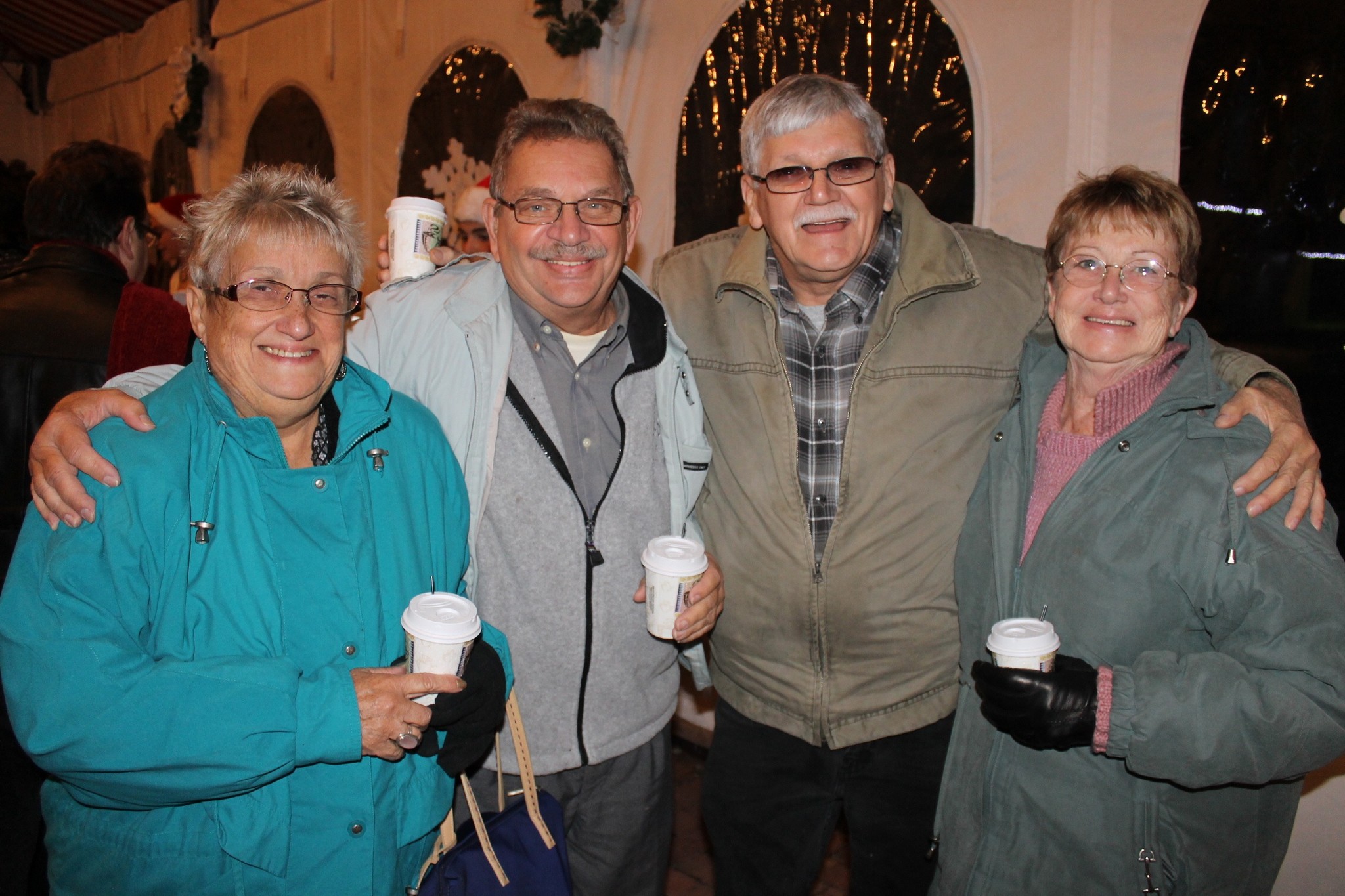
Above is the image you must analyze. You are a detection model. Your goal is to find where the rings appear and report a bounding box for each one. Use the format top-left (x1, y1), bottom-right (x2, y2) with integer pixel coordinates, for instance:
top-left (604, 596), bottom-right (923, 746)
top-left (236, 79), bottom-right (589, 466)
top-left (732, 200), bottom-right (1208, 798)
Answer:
top-left (397, 725), bottom-right (419, 749)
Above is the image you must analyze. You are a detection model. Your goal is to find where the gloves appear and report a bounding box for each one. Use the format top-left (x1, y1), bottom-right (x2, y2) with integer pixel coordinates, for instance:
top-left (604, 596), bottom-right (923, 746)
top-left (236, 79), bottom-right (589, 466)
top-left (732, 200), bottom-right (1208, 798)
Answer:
top-left (970, 645), bottom-right (1099, 752)
top-left (389, 640), bottom-right (506, 777)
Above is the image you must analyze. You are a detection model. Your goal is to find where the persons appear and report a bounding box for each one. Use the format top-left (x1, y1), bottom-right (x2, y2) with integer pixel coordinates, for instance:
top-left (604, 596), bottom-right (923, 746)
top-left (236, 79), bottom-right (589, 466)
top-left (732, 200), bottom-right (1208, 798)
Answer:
top-left (931, 170), bottom-right (1344, 896)
top-left (453, 175), bottom-right (493, 264)
top-left (377, 76), bottom-right (1330, 896)
top-left (27, 100), bottom-right (727, 896)
top-left (0, 140), bottom-right (194, 587)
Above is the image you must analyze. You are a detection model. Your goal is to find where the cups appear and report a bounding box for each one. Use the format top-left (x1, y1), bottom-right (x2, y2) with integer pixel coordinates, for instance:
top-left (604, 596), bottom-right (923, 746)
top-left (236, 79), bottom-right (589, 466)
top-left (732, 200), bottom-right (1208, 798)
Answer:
top-left (400, 592), bottom-right (482, 706)
top-left (986, 617), bottom-right (1060, 672)
top-left (384, 197), bottom-right (447, 279)
top-left (640, 535), bottom-right (709, 639)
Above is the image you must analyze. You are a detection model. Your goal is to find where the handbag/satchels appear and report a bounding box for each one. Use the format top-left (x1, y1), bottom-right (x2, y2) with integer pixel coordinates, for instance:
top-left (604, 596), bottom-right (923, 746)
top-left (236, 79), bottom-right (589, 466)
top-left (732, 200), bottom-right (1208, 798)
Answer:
top-left (416, 686), bottom-right (575, 896)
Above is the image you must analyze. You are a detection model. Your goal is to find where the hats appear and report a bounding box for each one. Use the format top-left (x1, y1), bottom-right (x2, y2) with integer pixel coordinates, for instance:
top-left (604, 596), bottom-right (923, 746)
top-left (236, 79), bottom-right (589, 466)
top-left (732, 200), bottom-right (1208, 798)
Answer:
top-left (148, 192), bottom-right (207, 239)
top-left (454, 174), bottom-right (493, 223)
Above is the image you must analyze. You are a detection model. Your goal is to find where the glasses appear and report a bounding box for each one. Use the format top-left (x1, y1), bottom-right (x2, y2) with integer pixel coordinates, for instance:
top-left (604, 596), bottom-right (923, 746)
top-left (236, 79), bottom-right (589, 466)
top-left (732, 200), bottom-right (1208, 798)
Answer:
top-left (197, 278), bottom-right (362, 316)
top-left (1054, 254), bottom-right (1184, 293)
top-left (114, 221), bottom-right (162, 248)
top-left (498, 195), bottom-right (630, 226)
top-left (745, 151), bottom-right (888, 193)
